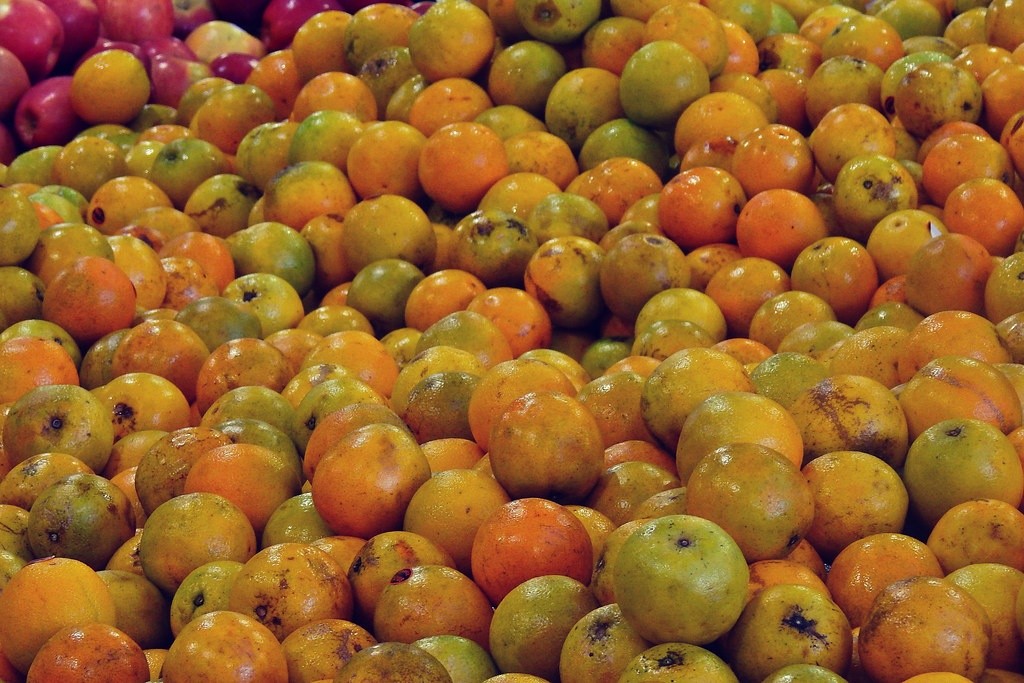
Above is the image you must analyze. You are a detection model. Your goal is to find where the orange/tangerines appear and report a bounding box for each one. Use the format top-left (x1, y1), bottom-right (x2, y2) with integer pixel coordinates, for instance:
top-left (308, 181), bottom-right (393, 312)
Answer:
top-left (404, 0), bottom-right (1024, 84)
top-left (0, 52), bottom-right (1024, 683)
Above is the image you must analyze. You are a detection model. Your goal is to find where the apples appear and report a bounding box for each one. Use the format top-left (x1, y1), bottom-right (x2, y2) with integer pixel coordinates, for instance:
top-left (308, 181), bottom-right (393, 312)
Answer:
top-left (0, 0), bottom-right (434, 162)
